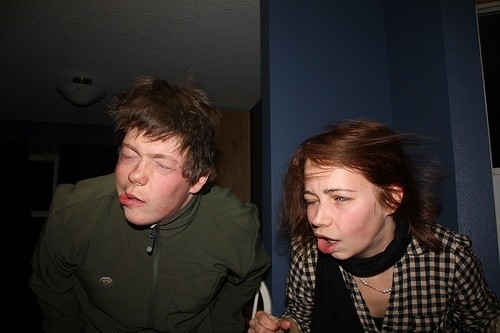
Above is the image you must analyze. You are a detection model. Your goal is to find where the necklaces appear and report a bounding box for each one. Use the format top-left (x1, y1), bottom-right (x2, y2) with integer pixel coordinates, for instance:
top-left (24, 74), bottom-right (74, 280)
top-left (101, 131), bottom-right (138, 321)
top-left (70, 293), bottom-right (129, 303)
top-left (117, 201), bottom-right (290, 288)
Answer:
top-left (357, 276), bottom-right (392, 293)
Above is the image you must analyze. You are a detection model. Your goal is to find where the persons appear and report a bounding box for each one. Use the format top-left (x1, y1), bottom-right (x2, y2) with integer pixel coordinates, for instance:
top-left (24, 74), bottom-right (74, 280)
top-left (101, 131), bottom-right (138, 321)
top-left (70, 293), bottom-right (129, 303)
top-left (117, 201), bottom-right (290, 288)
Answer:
top-left (246, 118), bottom-right (500, 333)
top-left (26, 73), bottom-right (272, 332)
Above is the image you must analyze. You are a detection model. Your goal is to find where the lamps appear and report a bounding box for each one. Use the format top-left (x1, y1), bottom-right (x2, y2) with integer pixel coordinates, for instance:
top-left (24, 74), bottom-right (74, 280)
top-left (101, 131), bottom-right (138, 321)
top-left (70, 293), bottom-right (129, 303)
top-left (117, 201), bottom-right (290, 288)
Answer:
top-left (55, 74), bottom-right (107, 106)
